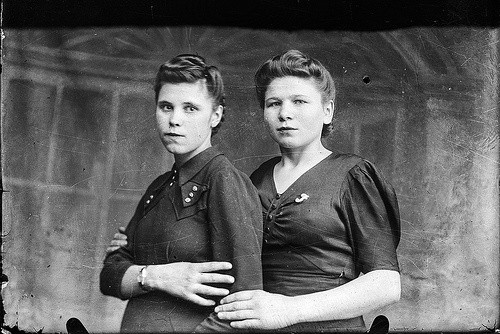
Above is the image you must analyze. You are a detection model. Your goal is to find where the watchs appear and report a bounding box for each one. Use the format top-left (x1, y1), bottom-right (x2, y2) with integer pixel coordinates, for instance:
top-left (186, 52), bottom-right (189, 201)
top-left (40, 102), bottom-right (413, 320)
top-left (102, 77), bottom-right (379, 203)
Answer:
top-left (138, 265), bottom-right (153, 292)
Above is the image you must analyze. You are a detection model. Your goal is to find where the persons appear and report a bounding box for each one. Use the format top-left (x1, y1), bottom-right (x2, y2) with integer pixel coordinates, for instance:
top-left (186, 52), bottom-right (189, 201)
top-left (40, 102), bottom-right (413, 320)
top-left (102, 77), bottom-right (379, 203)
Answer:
top-left (106, 49), bottom-right (401, 334)
top-left (99, 53), bottom-right (266, 334)
